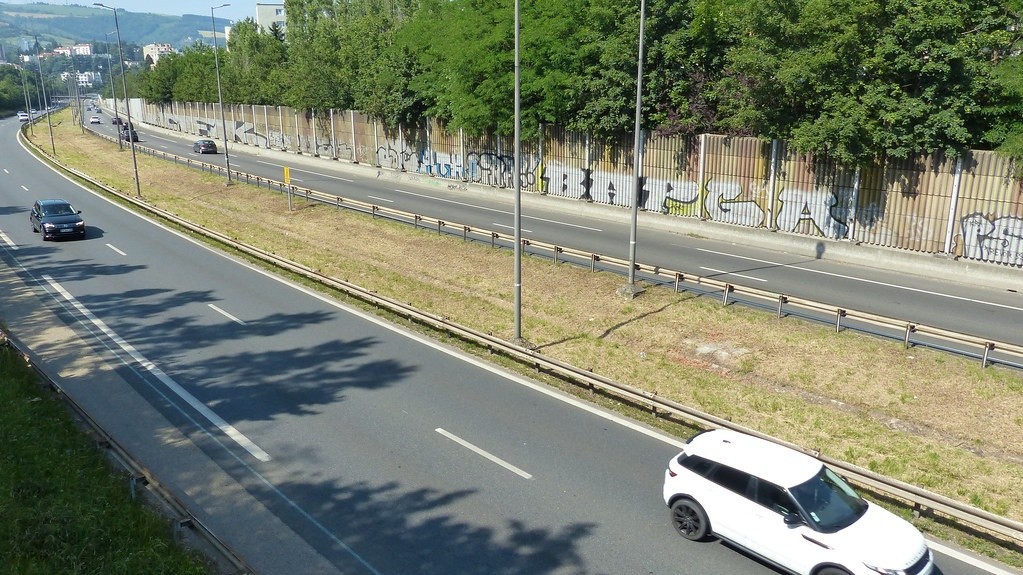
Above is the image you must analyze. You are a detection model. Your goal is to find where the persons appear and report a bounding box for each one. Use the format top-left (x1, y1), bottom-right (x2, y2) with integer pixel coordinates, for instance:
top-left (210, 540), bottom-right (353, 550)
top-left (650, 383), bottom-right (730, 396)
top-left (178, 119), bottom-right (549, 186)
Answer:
top-left (59, 206), bottom-right (69, 214)
top-left (41, 207), bottom-right (49, 214)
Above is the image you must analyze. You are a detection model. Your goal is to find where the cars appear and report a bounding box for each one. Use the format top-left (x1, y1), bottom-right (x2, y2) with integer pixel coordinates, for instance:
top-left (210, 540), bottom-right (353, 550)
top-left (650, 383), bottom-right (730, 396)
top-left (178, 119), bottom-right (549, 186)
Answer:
top-left (193, 140), bottom-right (218, 154)
top-left (121, 130), bottom-right (138, 142)
top-left (30, 199), bottom-right (86, 241)
top-left (17, 111), bottom-right (25, 116)
top-left (96, 109), bottom-right (102, 113)
top-left (112, 118), bottom-right (122, 125)
top-left (30, 109), bottom-right (37, 114)
top-left (122, 122), bottom-right (133, 130)
top-left (19, 114), bottom-right (28, 122)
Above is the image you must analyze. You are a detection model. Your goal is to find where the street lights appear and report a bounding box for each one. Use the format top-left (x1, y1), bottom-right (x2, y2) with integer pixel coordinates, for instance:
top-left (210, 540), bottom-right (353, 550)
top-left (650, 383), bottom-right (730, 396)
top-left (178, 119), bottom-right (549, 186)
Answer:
top-left (92, 3), bottom-right (140, 197)
top-left (211, 4), bottom-right (231, 184)
top-left (104, 31), bottom-right (122, 150)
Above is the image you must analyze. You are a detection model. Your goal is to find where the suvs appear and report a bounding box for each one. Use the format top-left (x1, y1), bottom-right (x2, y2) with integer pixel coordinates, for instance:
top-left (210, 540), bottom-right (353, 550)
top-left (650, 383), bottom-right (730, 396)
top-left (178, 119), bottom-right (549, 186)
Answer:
top-left (662, 426), bottom-right (934, 575)
top-left (90, 116), bottom-right (100, 124)
